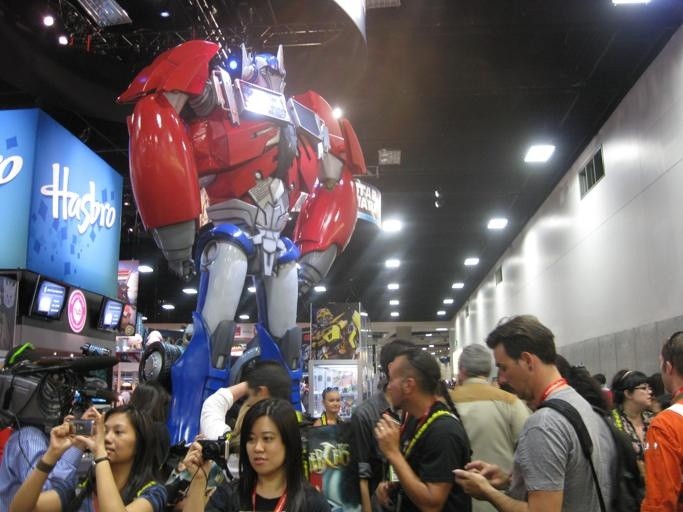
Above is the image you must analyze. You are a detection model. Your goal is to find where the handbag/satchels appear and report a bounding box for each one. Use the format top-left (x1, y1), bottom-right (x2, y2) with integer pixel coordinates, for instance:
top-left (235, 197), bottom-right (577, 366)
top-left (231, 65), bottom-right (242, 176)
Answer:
top-left (607, 418), bottom-right (647, 512)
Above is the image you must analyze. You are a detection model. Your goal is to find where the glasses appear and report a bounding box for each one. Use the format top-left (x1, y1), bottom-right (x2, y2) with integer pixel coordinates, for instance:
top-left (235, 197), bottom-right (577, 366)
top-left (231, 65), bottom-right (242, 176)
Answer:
top-left (636, 386), bottom-right (653, 391)
top-left (667, 331), bottom-right (683, 364)
top-left (324, 386), bottom-right (339, 395)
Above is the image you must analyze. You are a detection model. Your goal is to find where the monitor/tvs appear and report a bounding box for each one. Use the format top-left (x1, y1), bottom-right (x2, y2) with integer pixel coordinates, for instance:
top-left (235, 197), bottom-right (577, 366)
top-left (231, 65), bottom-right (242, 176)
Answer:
top-left (97, 295), bottom-right (122, 332)
top-left (120, 303), bottom-right (137, 336)
top-left (28, 276), bottom-right (70, 321)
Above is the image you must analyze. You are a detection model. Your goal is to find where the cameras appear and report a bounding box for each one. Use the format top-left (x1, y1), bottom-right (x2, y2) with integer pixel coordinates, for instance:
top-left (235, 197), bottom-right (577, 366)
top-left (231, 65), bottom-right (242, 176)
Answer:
top-left (197, 439), bottom-right (230, 459)
top-left (68, 420), bottom-right (95, 436)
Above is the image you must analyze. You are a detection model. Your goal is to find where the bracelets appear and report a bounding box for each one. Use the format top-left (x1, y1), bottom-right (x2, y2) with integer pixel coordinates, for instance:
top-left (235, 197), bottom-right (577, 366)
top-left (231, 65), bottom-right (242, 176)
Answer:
top-left (35, 458), bottom-right (57, 473)
top-left (94, 456), bottom-right (109, 464)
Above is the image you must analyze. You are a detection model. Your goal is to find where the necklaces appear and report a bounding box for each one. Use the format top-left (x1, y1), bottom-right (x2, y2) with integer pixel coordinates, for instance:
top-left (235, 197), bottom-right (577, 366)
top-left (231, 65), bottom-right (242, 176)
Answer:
top-left (624, 410), bottom-right (642, 420)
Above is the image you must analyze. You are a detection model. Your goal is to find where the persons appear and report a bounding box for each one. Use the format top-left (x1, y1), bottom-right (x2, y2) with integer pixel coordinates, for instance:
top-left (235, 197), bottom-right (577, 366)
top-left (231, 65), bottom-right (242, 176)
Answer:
top-left (375, 348), bottom-right (474, 512)
top-left (182, 397), bottom-right (333, 511)
top-left (122, 357), bottom-right (673, 481)
top-left (606, 369), bottom-right (658, 502)
top-left (313, 386), bottom-right (345, 427)
top-left (452, 314), bottom-right (619, 512)
top-left (0, 407), bottom-right (103, 511)
top-left (350, 338), bottom-right (417, 510)
top-left (639, 329), bottom-right (682, 512)
top-left (448, 343), bottom-right (530, 511)
top-left (8, 404), bottom-right (169, 511)
